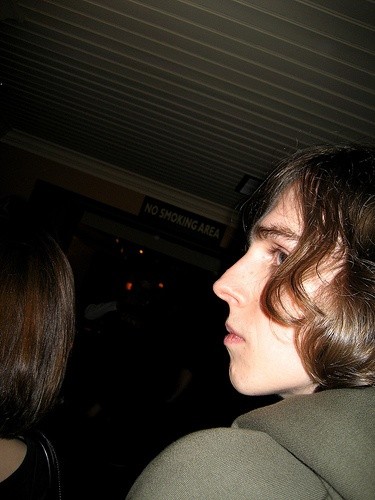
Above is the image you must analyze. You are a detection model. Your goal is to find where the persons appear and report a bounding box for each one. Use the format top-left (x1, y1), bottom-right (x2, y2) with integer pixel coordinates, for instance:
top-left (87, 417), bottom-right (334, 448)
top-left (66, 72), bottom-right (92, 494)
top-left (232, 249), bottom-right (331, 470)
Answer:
top-left (123, 143), bottom-right (375, 499)
top-left (0, 219), bottom-right (79, 500)
